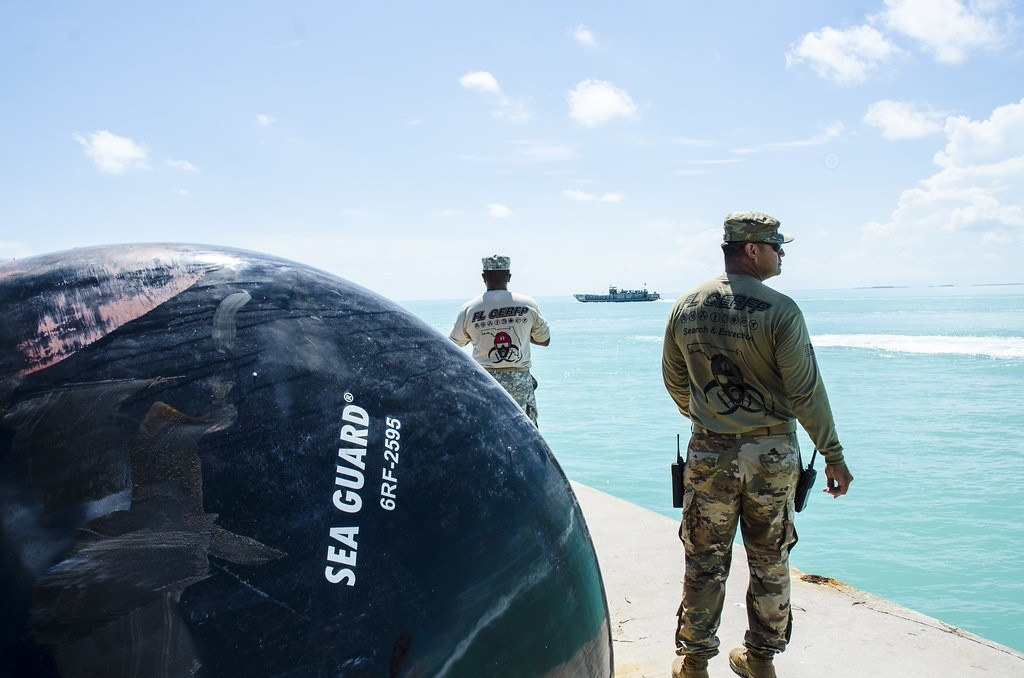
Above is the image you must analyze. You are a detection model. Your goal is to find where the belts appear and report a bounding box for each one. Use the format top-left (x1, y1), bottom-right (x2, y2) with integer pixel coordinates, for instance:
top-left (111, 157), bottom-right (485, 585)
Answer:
top-left (484, 367), bottom-right (529, 372)
top-left (692, 420), bottom-right (796, 436)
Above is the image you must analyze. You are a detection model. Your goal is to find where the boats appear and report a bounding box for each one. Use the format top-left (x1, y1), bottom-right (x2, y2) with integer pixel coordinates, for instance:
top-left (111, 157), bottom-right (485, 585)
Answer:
top-left (573, 286), bottom-right (661, 302)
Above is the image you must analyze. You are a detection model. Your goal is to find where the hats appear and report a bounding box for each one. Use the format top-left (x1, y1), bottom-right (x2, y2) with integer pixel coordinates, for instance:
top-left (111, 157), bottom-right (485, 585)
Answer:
top-left (723, 212), bottom-right (794, 244)
top-left (482, 254), bottom-right (511, 270)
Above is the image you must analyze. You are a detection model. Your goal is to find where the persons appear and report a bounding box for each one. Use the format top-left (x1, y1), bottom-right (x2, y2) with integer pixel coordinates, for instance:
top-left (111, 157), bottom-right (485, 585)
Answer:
top-left (447, 257), bottom-right (550, 431)
top-left (662, 209), bottom-right (853, 678)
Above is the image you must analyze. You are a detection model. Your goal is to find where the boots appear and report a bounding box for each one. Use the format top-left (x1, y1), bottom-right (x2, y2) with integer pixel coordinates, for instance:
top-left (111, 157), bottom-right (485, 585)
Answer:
top-left (728, 646), bottom-right (777, 678)
top-left (671, 649), bottom-right (709, 678)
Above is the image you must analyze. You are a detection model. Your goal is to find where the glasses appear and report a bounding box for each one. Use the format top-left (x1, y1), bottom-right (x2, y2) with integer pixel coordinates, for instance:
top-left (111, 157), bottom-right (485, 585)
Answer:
top-left (741, 240), bottom-right (782, 253)
